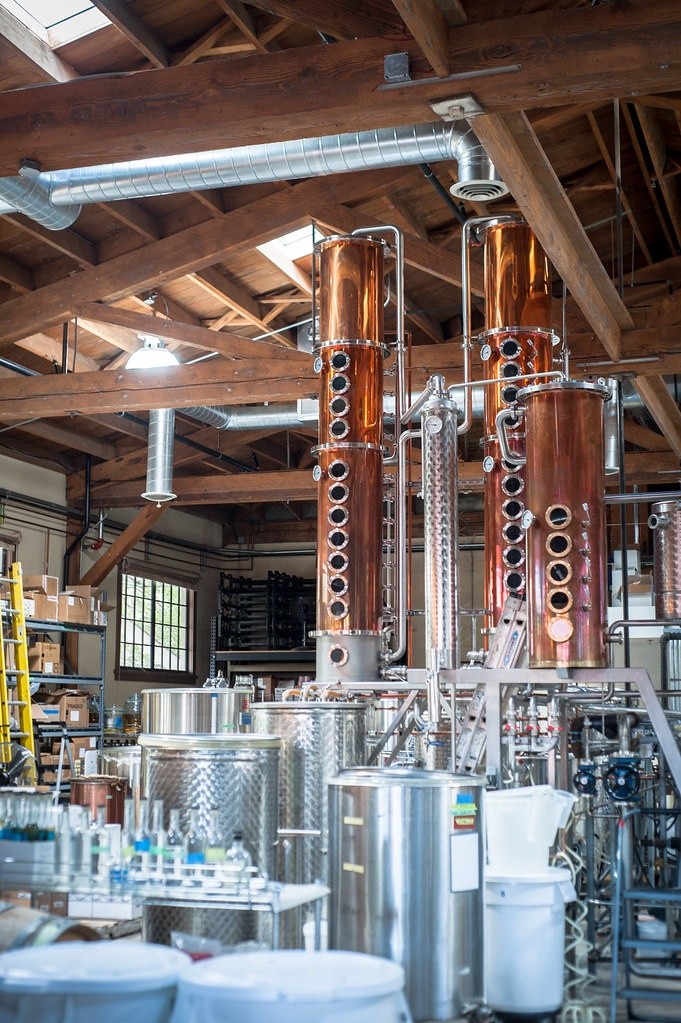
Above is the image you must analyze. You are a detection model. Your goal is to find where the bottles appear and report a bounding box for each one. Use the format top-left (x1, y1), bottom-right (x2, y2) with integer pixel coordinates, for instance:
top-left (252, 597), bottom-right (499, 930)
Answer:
top-left (90, 691), bottom-right (142, 734)
top-left (631, 719), bottom-right (681, 740)
top-left (297, 692), bottom-right (359, 703)
top-left (0, 790), bottom-right (252, 889)
top-left (202, 669), bottom-right (311, 703)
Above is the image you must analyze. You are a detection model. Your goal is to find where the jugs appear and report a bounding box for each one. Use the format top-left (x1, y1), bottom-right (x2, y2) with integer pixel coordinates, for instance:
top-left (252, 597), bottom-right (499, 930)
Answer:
top-left (527, 788), bottom-right (579, 847)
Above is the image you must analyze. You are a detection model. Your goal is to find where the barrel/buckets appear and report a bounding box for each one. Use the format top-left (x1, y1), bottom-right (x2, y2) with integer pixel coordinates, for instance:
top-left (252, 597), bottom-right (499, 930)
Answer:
top-left (0, 940), bottom-right (192, 1023)
top-left (168, 949), bottom-right (412, 1023)
top-left (140, 686), bottom-right (252, 735)
top-left (482, 866), bottom-right (577, 1014)
top-left (482, 784), bottom-right (554, 872)
top-left (329, 764), bottom-right (482, 1018)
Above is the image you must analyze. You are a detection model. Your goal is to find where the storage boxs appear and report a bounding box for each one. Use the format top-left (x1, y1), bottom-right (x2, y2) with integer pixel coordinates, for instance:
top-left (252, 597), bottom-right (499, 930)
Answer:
top-left (4, 627), bottom-right (98, 784)
top-left (0, 572), bottom-right (117, 625)
